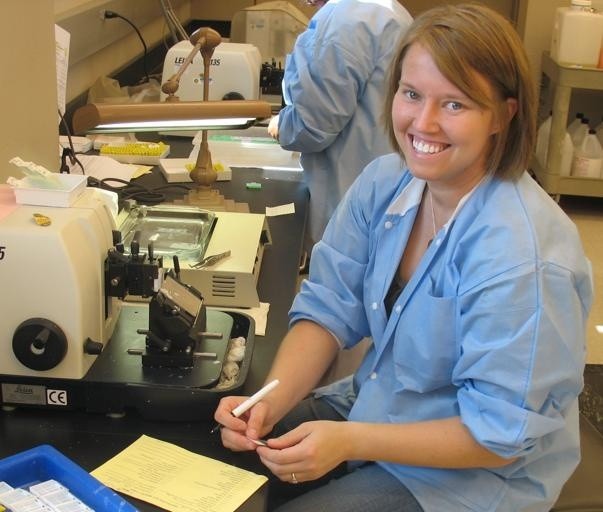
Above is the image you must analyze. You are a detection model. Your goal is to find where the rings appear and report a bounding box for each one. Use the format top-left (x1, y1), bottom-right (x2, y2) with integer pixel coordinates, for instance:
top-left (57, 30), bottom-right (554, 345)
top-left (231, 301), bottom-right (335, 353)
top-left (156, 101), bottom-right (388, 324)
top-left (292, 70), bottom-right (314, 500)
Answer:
top-left (291, 472), bottom-right (299, 485)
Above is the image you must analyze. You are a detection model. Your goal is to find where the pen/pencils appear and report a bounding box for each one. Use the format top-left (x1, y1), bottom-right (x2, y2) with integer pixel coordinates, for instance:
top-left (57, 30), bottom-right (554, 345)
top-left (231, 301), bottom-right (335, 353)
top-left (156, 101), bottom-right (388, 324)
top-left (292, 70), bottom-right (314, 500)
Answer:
top-left (212, 379), bottom-right (278, 435)
top-left (264, 58), bottom-right (283, 71)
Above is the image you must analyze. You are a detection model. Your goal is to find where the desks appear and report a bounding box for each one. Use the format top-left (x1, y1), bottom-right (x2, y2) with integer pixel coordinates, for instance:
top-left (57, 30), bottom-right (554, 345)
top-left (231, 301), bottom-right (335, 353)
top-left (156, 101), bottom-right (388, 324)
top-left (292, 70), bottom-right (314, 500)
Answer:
top-left (0, 131), bottom-right (310, 511)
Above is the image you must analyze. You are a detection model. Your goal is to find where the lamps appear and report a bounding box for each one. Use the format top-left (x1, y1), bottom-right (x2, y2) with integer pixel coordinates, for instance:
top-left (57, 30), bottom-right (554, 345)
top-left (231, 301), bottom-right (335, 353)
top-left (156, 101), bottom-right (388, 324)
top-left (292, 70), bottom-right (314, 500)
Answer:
top-left (72, 27), bottom-right (271, 214)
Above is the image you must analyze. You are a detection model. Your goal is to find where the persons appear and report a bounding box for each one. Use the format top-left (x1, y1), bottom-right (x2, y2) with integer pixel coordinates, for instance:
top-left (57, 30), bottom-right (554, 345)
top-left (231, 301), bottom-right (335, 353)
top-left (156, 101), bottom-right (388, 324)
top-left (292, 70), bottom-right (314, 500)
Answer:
top-left (212, 3), bottom-right (595, 512)
top-left (266, 0), bottom-right (417, 272)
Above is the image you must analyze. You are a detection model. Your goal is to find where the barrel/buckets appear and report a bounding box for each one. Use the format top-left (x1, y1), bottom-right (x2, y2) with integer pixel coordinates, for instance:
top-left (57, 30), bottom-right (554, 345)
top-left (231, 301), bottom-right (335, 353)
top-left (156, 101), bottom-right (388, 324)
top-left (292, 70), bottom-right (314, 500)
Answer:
top-left (536, 111), bottom-right (602, 178)
